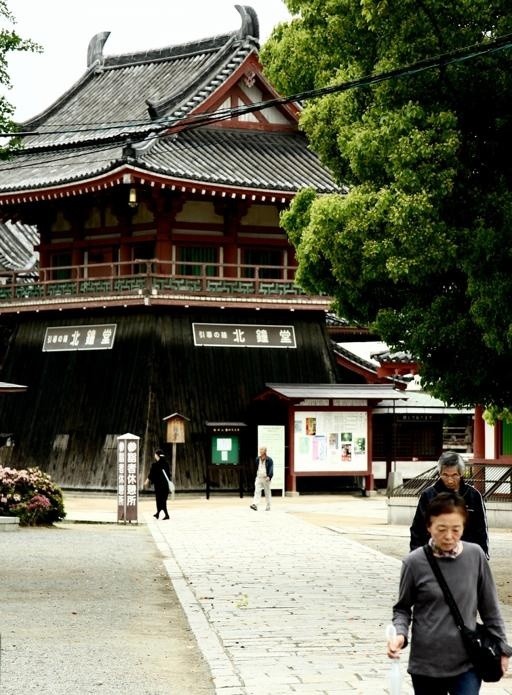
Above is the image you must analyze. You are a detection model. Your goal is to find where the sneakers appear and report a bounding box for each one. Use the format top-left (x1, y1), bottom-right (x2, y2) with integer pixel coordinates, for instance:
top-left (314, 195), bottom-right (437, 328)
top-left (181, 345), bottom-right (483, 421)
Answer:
top-left (250, 504), bottom-right (270, 511)
top-left (153, 514), bottom-right (170, 520)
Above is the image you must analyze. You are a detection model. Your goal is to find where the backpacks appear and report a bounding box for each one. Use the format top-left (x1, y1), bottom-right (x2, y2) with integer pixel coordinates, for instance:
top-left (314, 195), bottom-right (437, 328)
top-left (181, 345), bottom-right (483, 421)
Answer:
top-left (462, 619), bottom-right (504, 683)
top-left (169, 480), bottom-right (177, 495)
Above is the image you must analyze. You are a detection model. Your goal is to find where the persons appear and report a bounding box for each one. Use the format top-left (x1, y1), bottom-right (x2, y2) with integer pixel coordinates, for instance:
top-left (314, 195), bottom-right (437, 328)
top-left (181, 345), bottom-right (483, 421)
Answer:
top-left (250, 447), bottom-right (273, 510)
top-left (387, 451), bottom-right (512, 695)
top-left (144, 449), bottom-right (170, 520)
